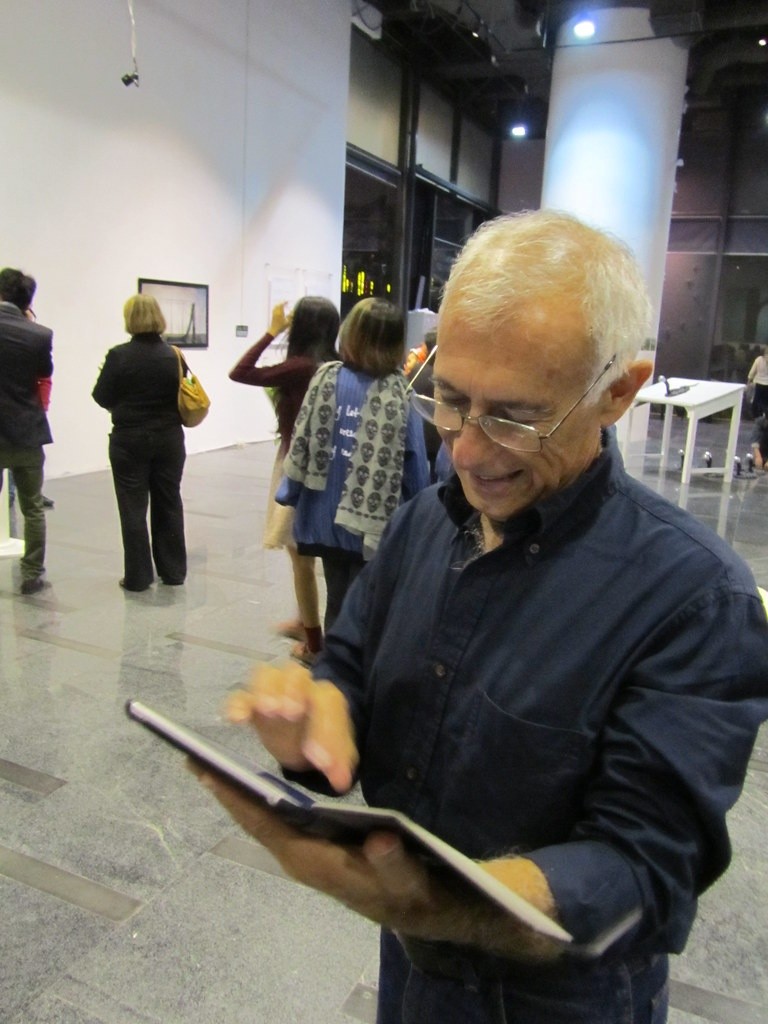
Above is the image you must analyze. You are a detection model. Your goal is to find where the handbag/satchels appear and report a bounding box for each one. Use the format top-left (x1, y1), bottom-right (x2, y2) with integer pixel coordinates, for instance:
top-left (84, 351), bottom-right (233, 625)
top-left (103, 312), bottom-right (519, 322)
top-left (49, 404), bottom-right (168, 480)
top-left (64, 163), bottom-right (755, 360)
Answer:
top-left (171, 345), bottom-right (211, 428)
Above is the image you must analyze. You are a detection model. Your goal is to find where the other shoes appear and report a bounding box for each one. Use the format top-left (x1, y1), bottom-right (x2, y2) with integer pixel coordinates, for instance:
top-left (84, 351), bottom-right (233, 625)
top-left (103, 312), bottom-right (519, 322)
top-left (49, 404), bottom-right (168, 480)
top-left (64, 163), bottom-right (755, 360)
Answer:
top-left (9, 497), bottom-right (13, 508)
top-left (43, 496), bottom-right (54, 506)
top-left (21, 579), bottom-right (44, 594)
top-left (119, 577), bottom-right (149, 592)
top-left (290, 643), bottom-right (315, 663)
top-left (162, 576), bottom-right (184, 585)
top-left (275, 622), bottom-right (306, 639)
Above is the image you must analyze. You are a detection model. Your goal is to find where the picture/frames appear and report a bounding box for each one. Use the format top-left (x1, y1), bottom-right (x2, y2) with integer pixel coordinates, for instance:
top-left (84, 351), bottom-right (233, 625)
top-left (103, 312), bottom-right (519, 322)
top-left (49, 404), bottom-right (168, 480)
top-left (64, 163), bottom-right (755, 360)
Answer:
top-left (136, 277), bottom-right (212, 348)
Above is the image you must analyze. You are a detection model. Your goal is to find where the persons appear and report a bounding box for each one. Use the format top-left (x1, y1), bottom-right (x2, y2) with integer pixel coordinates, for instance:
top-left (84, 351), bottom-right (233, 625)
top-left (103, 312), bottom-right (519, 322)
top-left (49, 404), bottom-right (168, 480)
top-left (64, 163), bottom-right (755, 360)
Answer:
top-left (185, 211), bottom-right (768, 1024)
top-left (746, 343), bottom-right (768, 470)
top-left (0, 266), bottom-right (55, 593)
top-left (92, 293), bottom-right (188, 591)
top-left (229, 296), bottom-right (436, 665)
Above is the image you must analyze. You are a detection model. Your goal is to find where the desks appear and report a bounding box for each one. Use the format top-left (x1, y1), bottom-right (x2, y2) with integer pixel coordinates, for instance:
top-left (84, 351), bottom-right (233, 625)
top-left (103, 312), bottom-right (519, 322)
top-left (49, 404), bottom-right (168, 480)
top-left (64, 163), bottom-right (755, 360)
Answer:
top-left (629, 376), bottom-right (746, 489)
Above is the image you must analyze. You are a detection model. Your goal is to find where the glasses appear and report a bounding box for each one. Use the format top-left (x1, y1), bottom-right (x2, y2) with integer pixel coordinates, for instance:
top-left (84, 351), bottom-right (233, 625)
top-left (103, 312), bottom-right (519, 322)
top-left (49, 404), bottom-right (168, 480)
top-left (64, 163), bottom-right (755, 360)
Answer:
top-left (404, 345), bottom-right (624, 452)
